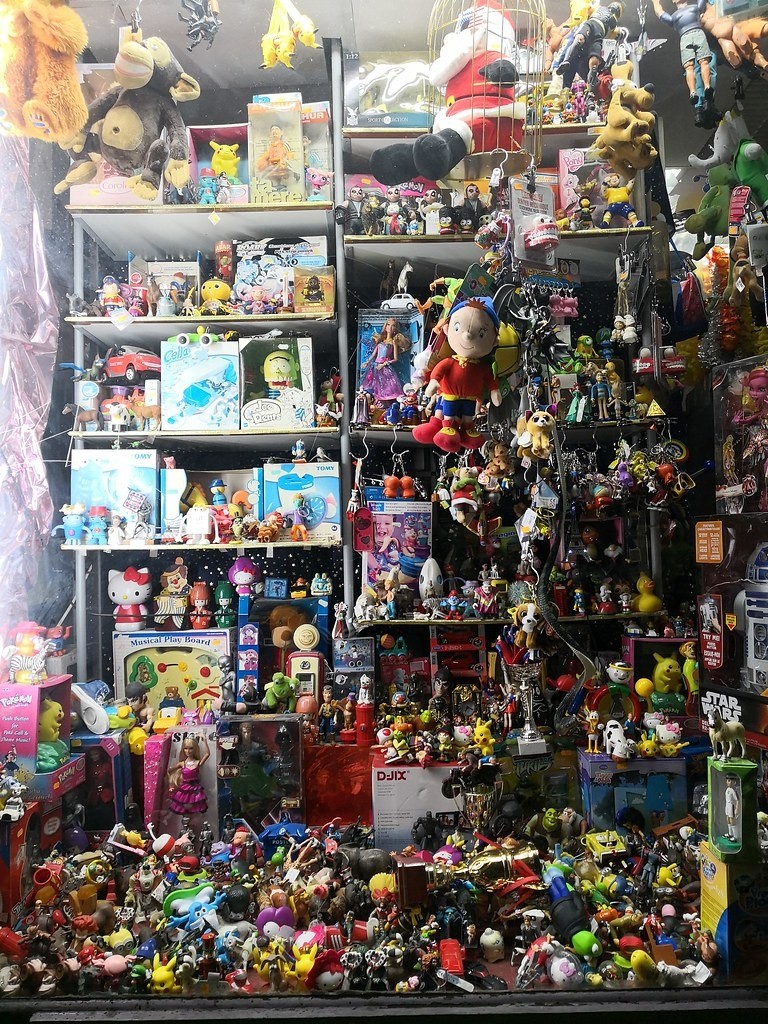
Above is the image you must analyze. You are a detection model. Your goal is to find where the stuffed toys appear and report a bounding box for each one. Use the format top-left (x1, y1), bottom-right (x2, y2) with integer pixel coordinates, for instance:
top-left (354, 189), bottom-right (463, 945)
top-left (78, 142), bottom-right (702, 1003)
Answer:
top-left (426, 296), bottom-right (502, 452)
top-left (0, 0), bottom-right (88, 143)
top-left (259, 0), bottom-right (324, 71)
top-left (537, 0), bottom-right (768, 307)
top-left (208, 140), bottom-right (241, 178)
top-left (369, 0), bottom-right (526, 186)
top-left (54, 36), bottom-right (200, 201)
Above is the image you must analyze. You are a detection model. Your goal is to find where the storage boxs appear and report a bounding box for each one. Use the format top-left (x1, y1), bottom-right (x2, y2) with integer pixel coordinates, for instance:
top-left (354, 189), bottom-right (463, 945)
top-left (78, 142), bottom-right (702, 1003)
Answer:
top-left (0, 91), bottom-right (768, 989)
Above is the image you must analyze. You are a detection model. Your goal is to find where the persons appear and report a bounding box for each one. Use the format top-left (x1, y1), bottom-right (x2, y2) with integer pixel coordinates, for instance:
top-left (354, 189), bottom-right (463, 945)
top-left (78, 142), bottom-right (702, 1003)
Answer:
top-left (556, 0), bottom-right (626, 85)
top-left (0, 184), bottom-right (768, 995)
top-left (253, 124), bottom-right (300, 180)
top-left (652, 0), bottom-right (714, 105)
top-left (599, 173), bottom-right (644, 229)
top-left (694, 34), bottom-right (723, 129)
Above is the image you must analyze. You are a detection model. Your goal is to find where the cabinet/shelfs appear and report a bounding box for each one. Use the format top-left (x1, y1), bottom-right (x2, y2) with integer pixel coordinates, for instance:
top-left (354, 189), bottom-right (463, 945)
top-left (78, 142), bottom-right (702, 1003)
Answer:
top-left (340, 122), bottom-right (662, 624)
top-left (69, 40), bottom-right (358, 719)
